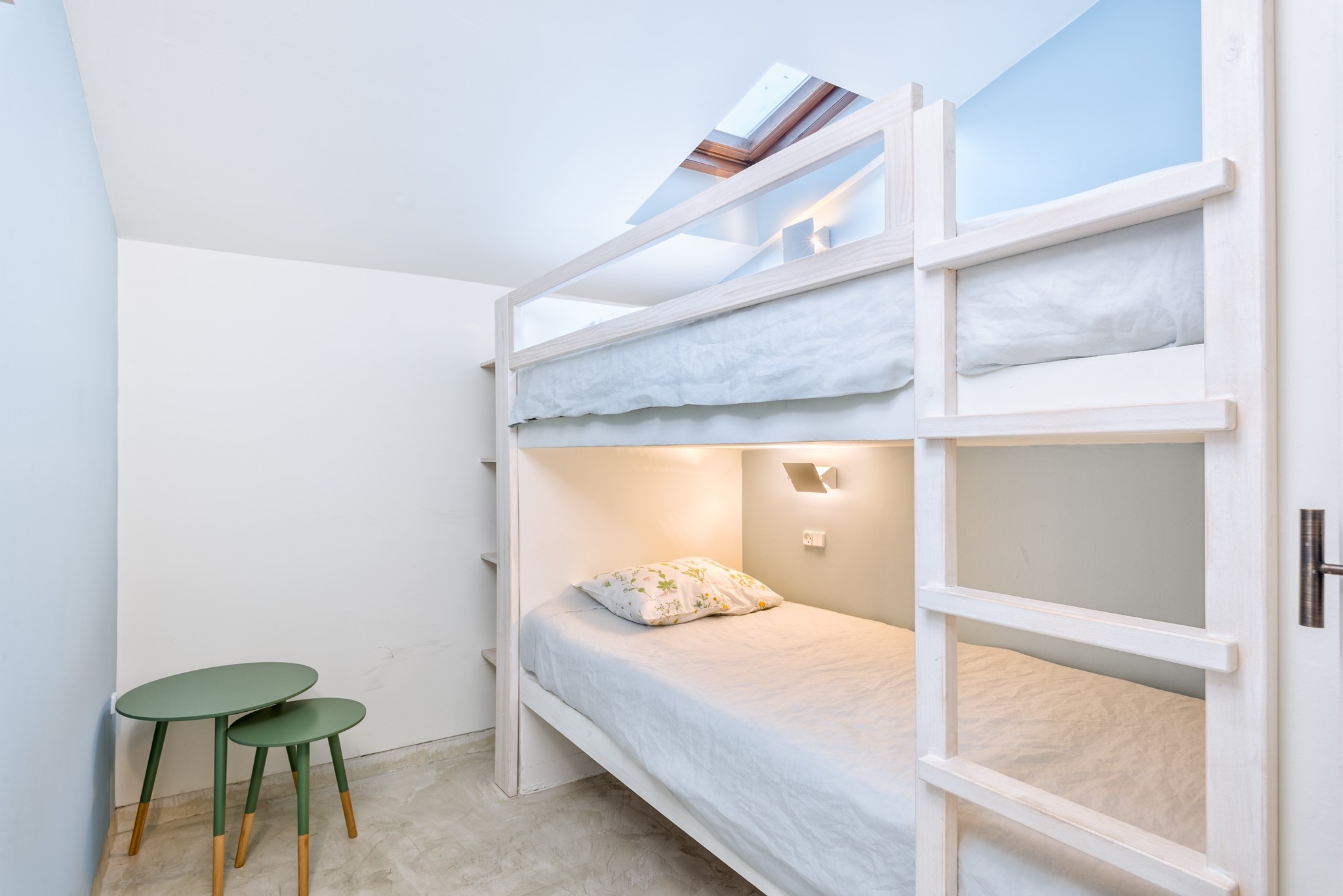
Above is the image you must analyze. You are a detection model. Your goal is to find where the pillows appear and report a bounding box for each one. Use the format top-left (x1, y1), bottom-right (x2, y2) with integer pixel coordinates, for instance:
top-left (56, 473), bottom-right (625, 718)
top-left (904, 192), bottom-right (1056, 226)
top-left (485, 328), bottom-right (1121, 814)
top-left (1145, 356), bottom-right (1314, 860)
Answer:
top-left (573, 557), bottom-right (784, 627)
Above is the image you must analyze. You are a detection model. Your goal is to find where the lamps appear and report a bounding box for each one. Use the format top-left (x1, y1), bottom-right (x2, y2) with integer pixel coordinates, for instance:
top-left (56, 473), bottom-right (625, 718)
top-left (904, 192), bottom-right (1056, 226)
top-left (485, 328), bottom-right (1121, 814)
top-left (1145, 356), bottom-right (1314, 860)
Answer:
top-left (783, 462), bottom-right (837, 493)
top-left (783, 217), bottom-right (830, 262)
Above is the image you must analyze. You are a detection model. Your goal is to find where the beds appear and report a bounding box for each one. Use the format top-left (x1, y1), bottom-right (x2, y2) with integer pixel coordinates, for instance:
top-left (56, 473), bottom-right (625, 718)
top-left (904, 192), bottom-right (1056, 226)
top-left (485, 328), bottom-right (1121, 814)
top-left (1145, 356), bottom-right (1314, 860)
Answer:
top-left (481, 0), bottom-right (1278, 896)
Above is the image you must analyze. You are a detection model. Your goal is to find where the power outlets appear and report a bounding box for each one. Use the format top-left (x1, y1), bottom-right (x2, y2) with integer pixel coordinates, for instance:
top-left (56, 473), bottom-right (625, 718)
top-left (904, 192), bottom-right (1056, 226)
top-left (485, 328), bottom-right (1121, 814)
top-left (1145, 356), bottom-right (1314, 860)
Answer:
top-left (803, 531), bottom-right (825, 548)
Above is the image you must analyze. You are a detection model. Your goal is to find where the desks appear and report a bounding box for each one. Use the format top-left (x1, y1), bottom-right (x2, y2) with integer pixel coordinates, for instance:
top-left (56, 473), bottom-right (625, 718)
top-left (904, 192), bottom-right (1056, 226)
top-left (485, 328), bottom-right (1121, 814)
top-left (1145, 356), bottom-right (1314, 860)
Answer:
top-left (115, 662), bottom-right (318, 896)
top-left (226, 698), bottom-right (366, 896)
